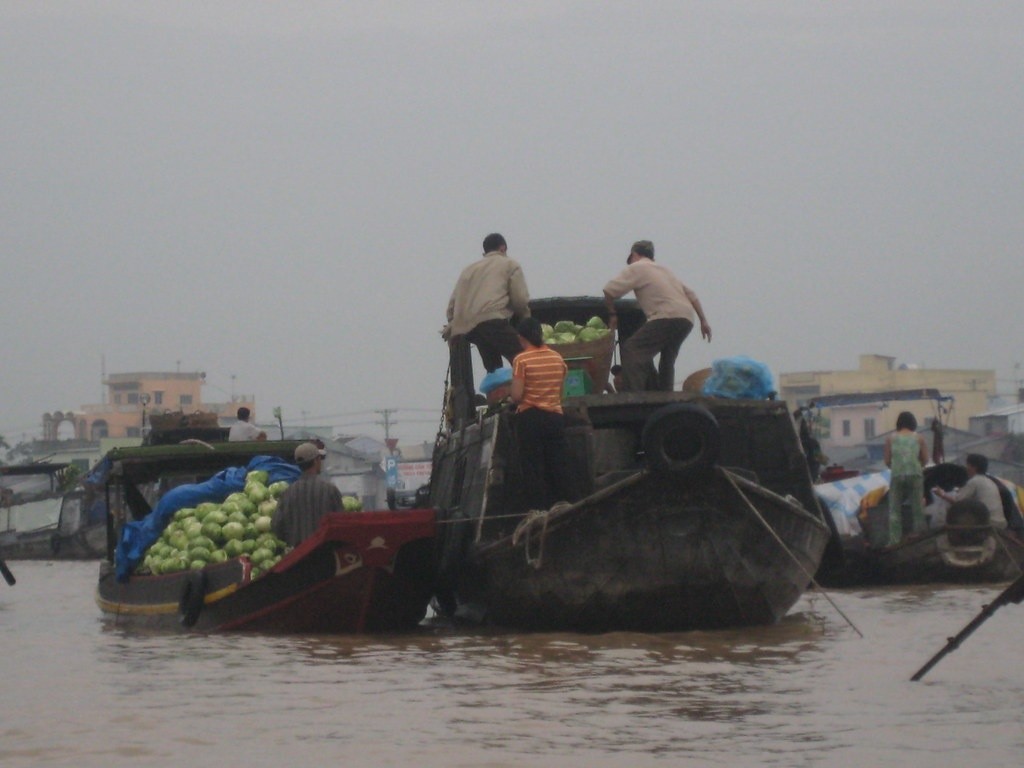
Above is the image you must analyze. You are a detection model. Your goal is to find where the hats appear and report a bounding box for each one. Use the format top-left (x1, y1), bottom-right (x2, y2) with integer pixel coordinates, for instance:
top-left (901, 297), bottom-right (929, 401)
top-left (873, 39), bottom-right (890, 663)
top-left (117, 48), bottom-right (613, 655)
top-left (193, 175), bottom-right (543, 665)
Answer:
top-left (627, 240), bottom-right (654, 264)
top-left (295, 443), bottom-right (327, 463)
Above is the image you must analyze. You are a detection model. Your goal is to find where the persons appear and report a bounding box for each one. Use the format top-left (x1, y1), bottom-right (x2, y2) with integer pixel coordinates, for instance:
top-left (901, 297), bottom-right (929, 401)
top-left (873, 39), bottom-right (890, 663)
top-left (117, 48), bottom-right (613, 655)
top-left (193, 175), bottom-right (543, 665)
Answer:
top-left (934, 453), bottom-right (1023, 531)
top-left (602, 241), bottom-right (712, 393)
top-left (511, 317), bottom-right (584, 509)
top-left (273, 443), bottom-right (344, 546)
top-left (229, 407), bottom-right (268, 441)
top-left (881, 412), bottom-right (928, 548)
top-left (442, 233), bottom-right (531, 373)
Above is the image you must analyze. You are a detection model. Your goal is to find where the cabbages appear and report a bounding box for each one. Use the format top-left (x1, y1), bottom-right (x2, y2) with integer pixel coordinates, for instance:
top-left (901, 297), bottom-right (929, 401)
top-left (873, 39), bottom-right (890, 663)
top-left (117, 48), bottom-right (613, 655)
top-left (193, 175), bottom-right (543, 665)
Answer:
top-left (539, 316), bottom-right (610, 343)
top-left (142, 469), bottom-right (361, 574)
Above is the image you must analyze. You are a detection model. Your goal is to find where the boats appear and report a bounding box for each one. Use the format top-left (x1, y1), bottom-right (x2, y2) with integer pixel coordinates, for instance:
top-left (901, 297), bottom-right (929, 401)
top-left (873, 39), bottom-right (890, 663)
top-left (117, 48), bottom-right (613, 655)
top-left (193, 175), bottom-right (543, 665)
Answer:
top-left (384, 296), bottom-right (831, 636)
top-left (82, 439), bottom-right (439, 636)
top-left (0, 463), bottom-right (70, 558)
top-left (853, 461), bottom-right (1024, 586)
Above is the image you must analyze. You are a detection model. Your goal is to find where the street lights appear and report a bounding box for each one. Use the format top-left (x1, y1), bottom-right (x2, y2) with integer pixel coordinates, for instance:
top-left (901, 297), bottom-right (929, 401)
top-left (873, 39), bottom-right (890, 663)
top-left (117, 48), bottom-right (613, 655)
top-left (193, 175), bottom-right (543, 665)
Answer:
top-left (140, 394), bottom-right (149, 434)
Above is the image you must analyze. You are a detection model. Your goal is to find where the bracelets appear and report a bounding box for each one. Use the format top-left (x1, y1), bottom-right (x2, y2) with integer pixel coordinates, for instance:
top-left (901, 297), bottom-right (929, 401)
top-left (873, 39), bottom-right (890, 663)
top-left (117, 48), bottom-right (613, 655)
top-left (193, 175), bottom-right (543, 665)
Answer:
top-left (942, 492), bottom-right (945, 497)
top-left (607, 311), bottom-right (618, 315)
